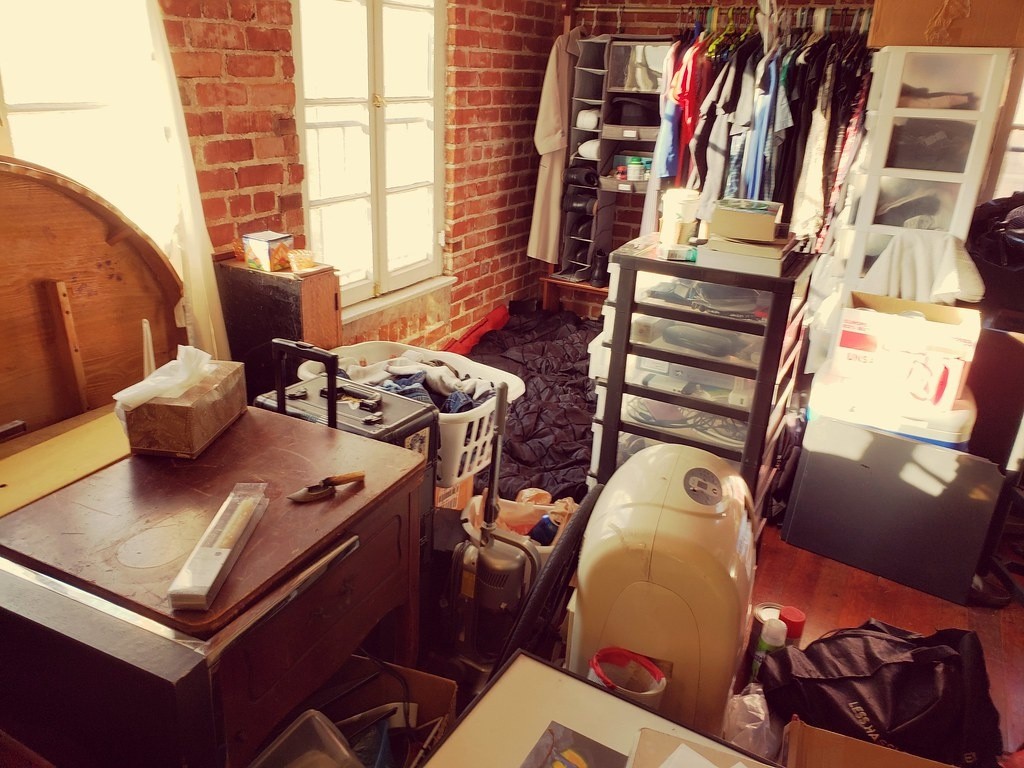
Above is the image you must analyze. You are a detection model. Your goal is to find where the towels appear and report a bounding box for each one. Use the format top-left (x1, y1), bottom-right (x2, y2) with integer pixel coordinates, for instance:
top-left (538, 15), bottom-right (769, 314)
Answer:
top-left (854, 228), bottom-right (986, 304)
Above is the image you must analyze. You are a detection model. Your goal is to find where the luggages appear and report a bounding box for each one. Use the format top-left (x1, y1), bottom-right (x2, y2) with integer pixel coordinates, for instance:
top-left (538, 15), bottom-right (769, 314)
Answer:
top-left (254, 337), bottom-right (440, 597)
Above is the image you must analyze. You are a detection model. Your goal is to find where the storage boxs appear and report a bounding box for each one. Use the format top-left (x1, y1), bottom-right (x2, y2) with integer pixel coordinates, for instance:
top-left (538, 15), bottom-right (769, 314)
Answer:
top-left (709, 197), bottom-right (783, 241)
top-left (435, 476), bottom-right (473, 510)
top-left (241, 231), bottom-right (294, 272)
top-left (822, 292), bottom-right (981, 413)
top-left (247, 708), bottom-right (366, 768)
top-left (866, 0), bottom-right (1024, 49)
top-left (782, 714), bottom-right (962, 768)
top-left (260, 653), bottom-right (457, 768)
top-left (806, 362), bottom-right (977, 454)
top-left (780, 329), bottom-right (1024, 605)
top-left (297, 340), bottom-right (526, 488)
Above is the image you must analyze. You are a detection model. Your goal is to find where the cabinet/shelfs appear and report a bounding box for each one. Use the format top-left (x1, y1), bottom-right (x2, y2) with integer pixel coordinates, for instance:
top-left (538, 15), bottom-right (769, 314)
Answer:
top-left (587, 233), bottom-right (819, 566)
top-left (540, 34), bottom-right (674, 311)
top-left (843, 44), bottom-right (1012, 287)
top-left (217, 258), bottom-right (342, 396)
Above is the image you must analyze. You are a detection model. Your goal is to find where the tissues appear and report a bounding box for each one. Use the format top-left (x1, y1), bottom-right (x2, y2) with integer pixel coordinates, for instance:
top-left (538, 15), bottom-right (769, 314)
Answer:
top-left (111, 342), bottom-right (248, 460)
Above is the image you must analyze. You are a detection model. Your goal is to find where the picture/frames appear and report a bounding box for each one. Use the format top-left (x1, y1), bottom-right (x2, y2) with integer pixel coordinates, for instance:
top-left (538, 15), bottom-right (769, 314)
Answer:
top-left (417, 648), bottom-right (786, 768)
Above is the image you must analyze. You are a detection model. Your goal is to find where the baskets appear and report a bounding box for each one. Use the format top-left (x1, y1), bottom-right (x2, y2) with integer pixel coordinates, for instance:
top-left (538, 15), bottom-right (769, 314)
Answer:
top-left (298, 340), bottom-right (526, 488)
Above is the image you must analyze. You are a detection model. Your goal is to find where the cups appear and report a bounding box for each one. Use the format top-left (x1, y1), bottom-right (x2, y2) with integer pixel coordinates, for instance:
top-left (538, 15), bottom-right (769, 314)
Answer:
top-left (662, 188), bottom-right (701, 223)
top-left (289, 249), bottom-right (314, 271)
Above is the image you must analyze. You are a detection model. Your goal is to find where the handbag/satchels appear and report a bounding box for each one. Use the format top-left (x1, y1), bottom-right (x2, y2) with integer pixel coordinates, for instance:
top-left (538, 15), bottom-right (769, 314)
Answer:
top-left (757, 618), bottom-right (1004, 768)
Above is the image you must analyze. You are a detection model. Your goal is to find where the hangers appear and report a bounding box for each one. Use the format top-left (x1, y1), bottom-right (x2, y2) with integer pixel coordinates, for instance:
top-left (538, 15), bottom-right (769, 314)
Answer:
top-left (580, 6), bottom-right (589, 39)
top-left (672, 6), bottom-right (871, 68)
top-left (616, 6), bottom-right (623, 35)
top-left (589, 7), bottom-right (600, 38)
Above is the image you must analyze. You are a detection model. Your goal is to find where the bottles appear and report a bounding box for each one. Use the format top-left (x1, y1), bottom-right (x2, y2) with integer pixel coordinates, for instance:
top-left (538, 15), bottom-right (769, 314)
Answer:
top-left (627, 157), bottom-right (645, 180)
top-left (750, 619), bottom-right (788, 683)
top-left (643, 164), bottom-right (651, 180)
top-left (615, 166), bottom-right (627, 181)
top-left (779, 606), bottom-right (806, 648)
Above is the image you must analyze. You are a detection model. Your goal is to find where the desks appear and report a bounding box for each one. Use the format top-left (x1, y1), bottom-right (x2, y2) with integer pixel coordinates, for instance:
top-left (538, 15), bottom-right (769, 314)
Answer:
top-left (1, 156), bottom-right (205, 445)
top-left (0, 403), bottom-right (426, 768)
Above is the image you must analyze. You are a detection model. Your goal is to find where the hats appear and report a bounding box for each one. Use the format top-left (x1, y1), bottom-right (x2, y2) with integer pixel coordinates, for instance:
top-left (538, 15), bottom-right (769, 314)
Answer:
top-left (612, 97), bottom-right (660, 126)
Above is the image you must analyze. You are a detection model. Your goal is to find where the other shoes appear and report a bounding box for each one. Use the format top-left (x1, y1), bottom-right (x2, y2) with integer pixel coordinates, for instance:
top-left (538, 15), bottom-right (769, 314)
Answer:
top-left (562, 109), bottom-right (610, 288)
